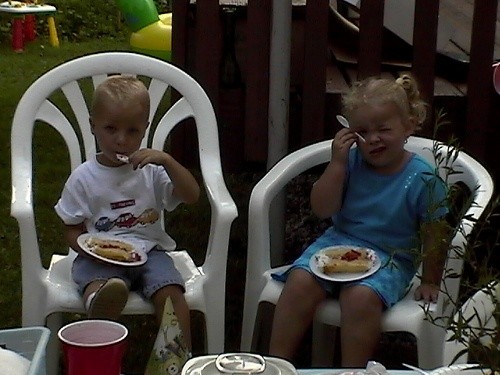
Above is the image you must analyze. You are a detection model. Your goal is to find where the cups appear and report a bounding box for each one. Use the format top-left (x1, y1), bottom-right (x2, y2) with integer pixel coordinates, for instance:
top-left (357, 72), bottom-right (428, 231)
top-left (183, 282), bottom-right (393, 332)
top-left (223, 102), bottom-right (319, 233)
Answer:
top-left (58, 320), bottom-right (128, 375)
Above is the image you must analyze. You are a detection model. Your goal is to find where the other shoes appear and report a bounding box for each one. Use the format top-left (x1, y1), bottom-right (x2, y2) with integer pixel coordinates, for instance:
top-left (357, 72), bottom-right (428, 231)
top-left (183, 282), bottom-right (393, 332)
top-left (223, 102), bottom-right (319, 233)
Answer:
top-left (88, 277), bottom-right (128, 321)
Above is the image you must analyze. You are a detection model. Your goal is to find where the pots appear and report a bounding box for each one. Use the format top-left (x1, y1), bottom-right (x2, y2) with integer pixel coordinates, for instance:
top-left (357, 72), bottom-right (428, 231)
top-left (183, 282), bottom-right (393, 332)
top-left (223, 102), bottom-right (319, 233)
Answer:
top-left (180, 353), bottom-right (299, 375)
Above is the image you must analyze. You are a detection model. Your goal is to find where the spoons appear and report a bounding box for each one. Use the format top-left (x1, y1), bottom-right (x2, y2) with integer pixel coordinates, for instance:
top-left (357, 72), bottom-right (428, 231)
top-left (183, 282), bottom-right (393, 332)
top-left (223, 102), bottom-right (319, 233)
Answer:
top-left (116, 154), bottom-right (158, 168)
top-left (337, 115), bottom-right (366, 143)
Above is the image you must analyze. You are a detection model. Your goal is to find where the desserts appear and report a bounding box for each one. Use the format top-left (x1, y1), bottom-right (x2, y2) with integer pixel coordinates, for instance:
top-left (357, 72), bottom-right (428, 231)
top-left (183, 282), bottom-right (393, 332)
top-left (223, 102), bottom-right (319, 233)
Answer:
top-left (87, 238), bottom-right (133, 261)
top-left (321, 247), bottom-right (369, 274)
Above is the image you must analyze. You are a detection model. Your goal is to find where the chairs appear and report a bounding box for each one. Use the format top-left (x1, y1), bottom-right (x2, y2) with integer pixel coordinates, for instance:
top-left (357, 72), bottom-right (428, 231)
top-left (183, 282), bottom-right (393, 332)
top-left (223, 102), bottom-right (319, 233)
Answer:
top-left (8, 51), bottom-right (240, 356)
top-left (239, 132), bottom-right (496, 369)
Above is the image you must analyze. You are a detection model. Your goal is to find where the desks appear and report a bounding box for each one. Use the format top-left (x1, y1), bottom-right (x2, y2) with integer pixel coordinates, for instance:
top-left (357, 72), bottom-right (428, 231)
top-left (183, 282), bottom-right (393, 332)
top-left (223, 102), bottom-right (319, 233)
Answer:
top-left (0, 0), bottom-right (61, 54)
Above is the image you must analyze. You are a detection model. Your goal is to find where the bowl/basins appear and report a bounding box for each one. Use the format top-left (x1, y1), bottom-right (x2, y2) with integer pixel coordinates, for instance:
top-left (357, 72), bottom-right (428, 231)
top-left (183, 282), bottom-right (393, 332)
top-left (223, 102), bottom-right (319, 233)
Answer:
top-left (0, 327), bottom-right (50, 375)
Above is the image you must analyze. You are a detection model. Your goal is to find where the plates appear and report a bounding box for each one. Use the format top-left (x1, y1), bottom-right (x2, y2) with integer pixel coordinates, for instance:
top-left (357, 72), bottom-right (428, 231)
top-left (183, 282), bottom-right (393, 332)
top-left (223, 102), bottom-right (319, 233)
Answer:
top-left (430, 363), bottom-right (499, 375)
top-left (77, 233), bottom-right (148, 266)
top-left (309, 246), bottom-right (382, 281)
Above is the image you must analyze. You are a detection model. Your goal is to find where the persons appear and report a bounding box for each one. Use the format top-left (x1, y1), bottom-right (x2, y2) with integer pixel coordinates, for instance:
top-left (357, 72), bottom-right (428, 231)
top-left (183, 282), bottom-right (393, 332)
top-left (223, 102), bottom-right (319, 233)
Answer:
top-left (269, 71), bottom-right (450, 368)
top-left (52, 75), bottom-right (201, 360)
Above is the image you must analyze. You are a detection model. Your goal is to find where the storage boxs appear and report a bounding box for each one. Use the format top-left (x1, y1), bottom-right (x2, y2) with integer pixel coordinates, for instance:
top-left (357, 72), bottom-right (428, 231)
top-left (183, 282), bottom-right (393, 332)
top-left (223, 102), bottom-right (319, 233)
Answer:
top-left (0, 326), bottom-right (51, 375)
